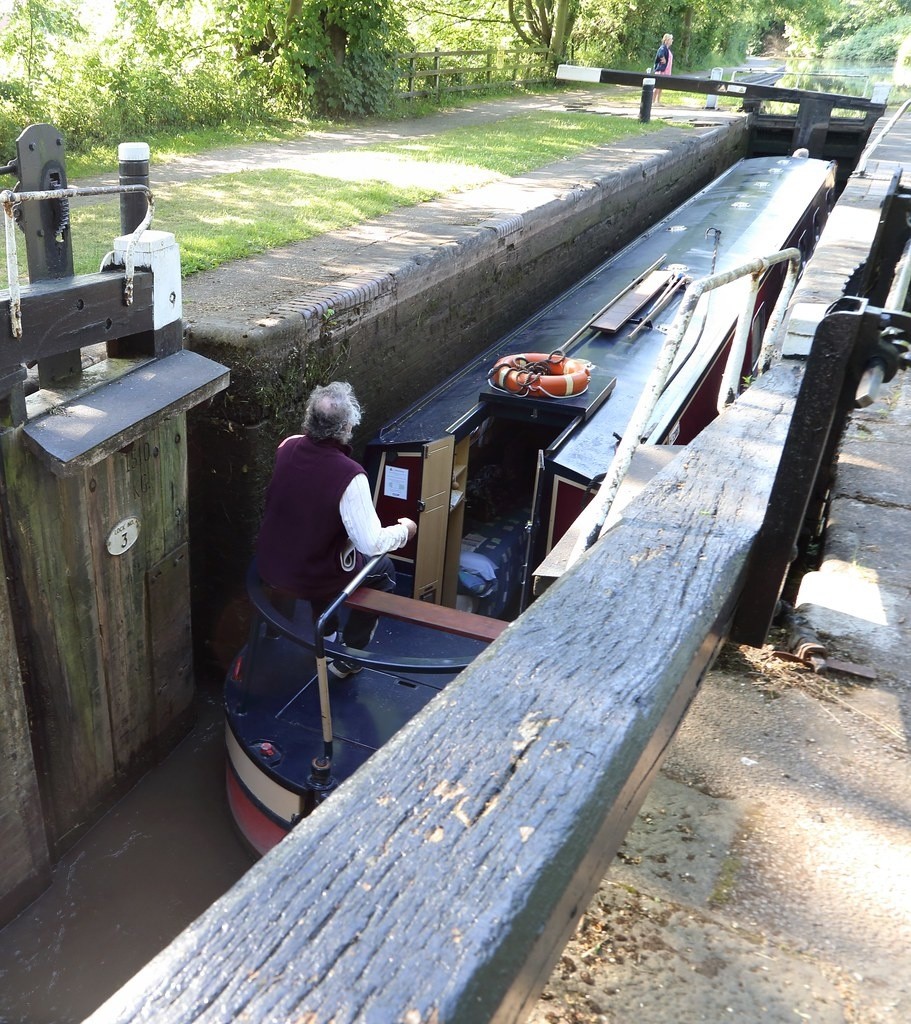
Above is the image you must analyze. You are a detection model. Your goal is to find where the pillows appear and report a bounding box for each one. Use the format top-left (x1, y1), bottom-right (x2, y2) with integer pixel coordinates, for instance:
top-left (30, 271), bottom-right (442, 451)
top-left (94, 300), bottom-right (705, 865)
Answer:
top-left (457, 552), bottom-right (499, 595)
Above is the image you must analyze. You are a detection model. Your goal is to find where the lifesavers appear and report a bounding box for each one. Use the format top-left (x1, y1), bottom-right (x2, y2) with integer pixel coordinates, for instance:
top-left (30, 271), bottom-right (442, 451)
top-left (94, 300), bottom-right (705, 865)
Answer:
top-left (493, 352), bottom-right (589, 398)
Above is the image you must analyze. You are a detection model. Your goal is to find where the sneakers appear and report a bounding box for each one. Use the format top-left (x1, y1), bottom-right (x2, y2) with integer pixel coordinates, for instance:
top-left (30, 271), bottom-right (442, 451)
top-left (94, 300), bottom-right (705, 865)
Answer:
top-left (328, 655), bottom-right (365, 679)
top-left (325, 633), bottom-right (348, 663)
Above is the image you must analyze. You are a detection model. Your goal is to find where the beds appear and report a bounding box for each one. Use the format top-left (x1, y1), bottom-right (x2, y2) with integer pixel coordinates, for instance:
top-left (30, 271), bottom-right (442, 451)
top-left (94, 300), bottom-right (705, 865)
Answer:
top-left (454, 456), bottom-right (542, 619)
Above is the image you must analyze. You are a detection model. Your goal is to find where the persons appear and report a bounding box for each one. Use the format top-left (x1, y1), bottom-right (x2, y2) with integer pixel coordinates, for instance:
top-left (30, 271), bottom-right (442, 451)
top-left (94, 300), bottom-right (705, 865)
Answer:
top-left (650, 34), bottom-right (673, 107)
top-left (254, 382), bottom-right (418, 677)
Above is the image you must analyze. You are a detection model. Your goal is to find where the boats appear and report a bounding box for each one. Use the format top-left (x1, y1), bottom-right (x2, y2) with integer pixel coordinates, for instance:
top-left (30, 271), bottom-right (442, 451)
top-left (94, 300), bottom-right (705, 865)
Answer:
top-left (222, 147), bottom-right (840, 862)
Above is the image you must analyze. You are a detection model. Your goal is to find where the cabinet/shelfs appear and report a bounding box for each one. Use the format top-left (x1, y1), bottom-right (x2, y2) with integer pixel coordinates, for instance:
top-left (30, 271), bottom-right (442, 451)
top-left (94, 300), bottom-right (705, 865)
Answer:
top-left (448, 437), bottom-right (470, 512)
